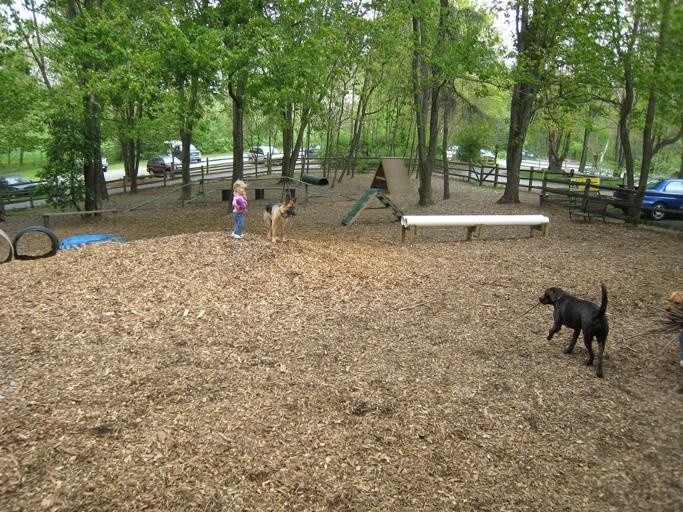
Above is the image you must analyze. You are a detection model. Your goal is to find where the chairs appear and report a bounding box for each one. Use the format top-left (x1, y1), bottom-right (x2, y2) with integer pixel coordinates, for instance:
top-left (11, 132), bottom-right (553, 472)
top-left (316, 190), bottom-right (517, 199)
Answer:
top-left (571, 195), bottom-right (607, 223)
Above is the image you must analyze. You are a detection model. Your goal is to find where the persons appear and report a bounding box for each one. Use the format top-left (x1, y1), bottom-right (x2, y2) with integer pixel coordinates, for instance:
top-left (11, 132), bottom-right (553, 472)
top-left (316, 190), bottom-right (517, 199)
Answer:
top-left (229, 179), bottom-right (249, 239)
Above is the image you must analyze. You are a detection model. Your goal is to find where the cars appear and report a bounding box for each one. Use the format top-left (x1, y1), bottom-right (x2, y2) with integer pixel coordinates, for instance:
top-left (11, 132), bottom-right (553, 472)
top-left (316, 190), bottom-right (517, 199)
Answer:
top-left (480, 149), bottom-right (495, 159)
top-left (0, 174), bottom-right (48, 197)
top-left (305, 142), bottom-right (321, 157)
top-left (100, 155), bottom-right (109, 171)
top-left (443, 144), bottom-right (459, 160)
top-left (161, 139), bottom-right (181, 159)
top-left (612, 178), bottom-right (682, 221)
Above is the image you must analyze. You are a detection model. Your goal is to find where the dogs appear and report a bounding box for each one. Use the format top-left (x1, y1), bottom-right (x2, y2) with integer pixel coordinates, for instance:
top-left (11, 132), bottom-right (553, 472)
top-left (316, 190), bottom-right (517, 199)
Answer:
top-left (262, 194), bottom-right (297, 243)
top-left (561, 168), bottom-right (574, 177)
top-left (538, 281), bottom-right (609, 378)
top-left (665, 290), bottom-right (683, 312)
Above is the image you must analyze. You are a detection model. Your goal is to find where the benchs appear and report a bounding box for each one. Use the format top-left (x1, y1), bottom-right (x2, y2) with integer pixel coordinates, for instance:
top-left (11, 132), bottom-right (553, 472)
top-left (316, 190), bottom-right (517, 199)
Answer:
top-left (215, 187), bottom-right (259, 202)
top-left (41, 208), bottom-right (116, 230)
top-left (261, 187), bottom-right (296, 202)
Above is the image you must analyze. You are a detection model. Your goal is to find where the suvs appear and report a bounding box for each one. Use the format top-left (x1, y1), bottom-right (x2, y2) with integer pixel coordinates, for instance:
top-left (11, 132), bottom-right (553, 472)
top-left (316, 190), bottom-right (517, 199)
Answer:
top-left (172, 144), bottom-right (202, 162)
top-left (247, 145), bottom-right (284, 162)
top-left (145, 154), bottom-right (184, 179)
